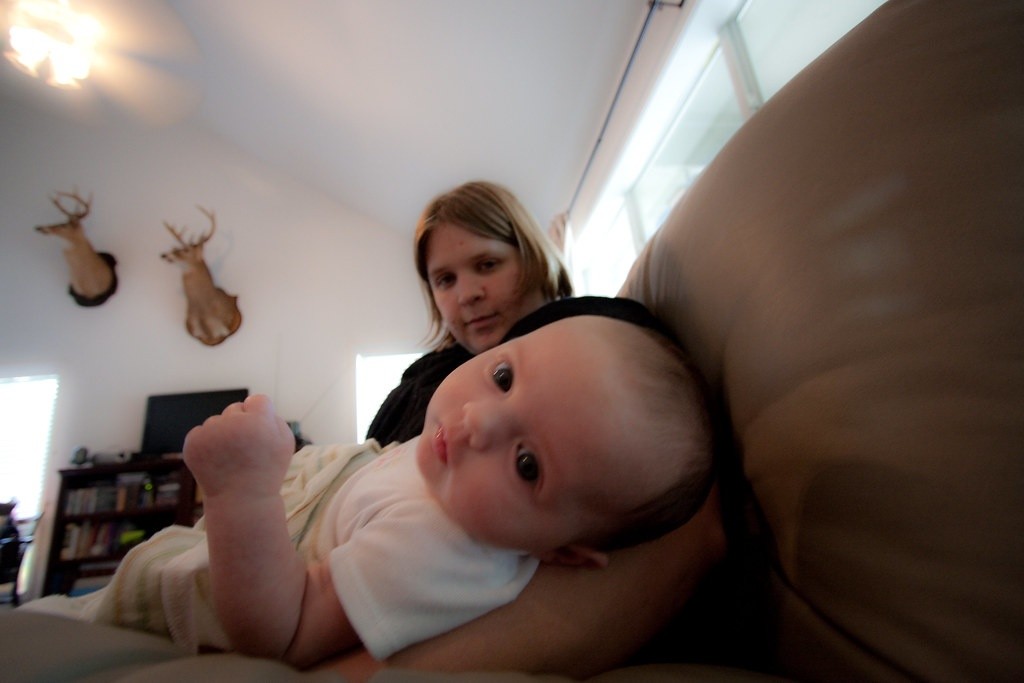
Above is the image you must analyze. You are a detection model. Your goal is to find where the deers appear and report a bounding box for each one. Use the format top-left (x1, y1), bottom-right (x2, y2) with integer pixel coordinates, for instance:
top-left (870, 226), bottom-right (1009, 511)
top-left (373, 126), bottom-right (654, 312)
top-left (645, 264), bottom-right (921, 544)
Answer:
top-left (34, 190), bottom-right (113, 297)
top-left (160, 205), bottom-right (241, 345)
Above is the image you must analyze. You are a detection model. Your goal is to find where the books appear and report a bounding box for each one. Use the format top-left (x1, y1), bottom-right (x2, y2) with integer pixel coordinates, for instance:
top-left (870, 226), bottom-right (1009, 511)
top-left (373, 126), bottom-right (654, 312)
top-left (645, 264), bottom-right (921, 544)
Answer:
top-left (65, 484), bottom-right (139, 516)
top-left (61, 520), bottom-right (116, 560)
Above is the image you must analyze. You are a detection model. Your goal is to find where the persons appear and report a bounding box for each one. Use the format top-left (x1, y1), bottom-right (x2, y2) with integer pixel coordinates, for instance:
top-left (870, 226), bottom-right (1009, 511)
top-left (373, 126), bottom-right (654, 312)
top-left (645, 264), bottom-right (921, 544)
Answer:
top-left (325, 181), bottom-right (776, 683)
top-left (15, 313), bottom-right (714, 671)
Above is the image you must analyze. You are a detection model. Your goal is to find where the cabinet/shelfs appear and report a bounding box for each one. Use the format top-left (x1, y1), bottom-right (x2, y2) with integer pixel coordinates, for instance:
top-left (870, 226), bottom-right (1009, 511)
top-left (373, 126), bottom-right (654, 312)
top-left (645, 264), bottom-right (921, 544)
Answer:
top-left (39, 458), bottom-right (203, 597)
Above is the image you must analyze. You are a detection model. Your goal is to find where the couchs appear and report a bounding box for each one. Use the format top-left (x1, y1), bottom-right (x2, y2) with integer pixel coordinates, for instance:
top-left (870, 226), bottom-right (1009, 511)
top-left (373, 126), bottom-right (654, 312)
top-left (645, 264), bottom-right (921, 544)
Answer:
top-left (0, 0), bottom-right (1024, 683)
